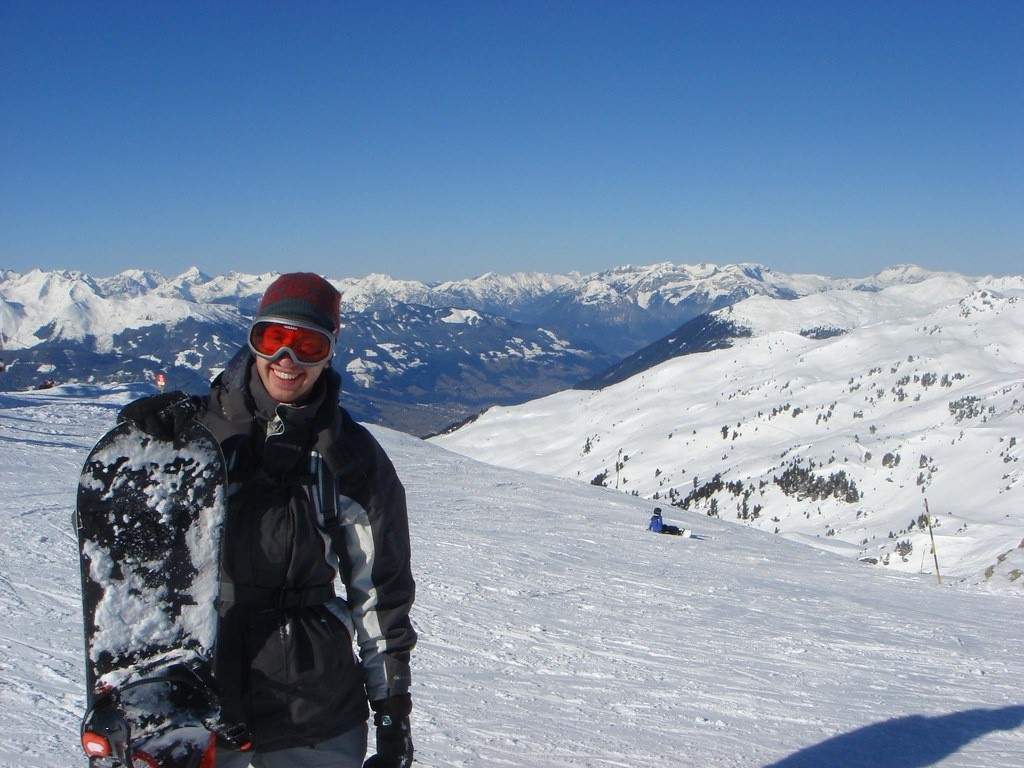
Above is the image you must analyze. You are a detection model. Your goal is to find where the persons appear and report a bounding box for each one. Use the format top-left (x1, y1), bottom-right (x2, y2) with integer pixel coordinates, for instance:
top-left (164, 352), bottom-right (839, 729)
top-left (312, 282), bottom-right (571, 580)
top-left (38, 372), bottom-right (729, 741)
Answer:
top-left (115, 273), bottom-right (416, 767)
top-left (646, 508), bottom-right (685, 535)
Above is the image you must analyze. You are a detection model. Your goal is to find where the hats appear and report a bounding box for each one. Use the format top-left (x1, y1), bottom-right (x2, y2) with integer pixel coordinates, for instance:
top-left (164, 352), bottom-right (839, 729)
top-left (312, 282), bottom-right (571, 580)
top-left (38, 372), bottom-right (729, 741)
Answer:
top-left (254, 272), bottom-right (341, 339)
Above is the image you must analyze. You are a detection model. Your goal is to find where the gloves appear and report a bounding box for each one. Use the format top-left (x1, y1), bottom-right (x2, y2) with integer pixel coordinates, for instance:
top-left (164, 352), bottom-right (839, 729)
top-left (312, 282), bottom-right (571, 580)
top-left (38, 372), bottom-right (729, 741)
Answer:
top-left (116, 390), bottom-right (202, 442)
top-left (362, 692), bottom-right (414, 768)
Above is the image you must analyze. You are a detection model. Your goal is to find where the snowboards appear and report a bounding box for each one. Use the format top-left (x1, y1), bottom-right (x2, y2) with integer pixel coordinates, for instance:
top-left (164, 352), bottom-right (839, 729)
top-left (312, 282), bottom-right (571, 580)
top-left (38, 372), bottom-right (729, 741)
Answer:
top-left (73, 415), bottom-right (229, 768)
top-left (679, 528), bottom-right (692, 538)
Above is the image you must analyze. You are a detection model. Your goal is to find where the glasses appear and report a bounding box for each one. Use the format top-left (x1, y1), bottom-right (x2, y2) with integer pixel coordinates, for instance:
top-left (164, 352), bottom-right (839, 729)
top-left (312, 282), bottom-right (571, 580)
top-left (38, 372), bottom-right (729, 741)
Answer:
top-left (247, 316), bottom-right (336, 367)
